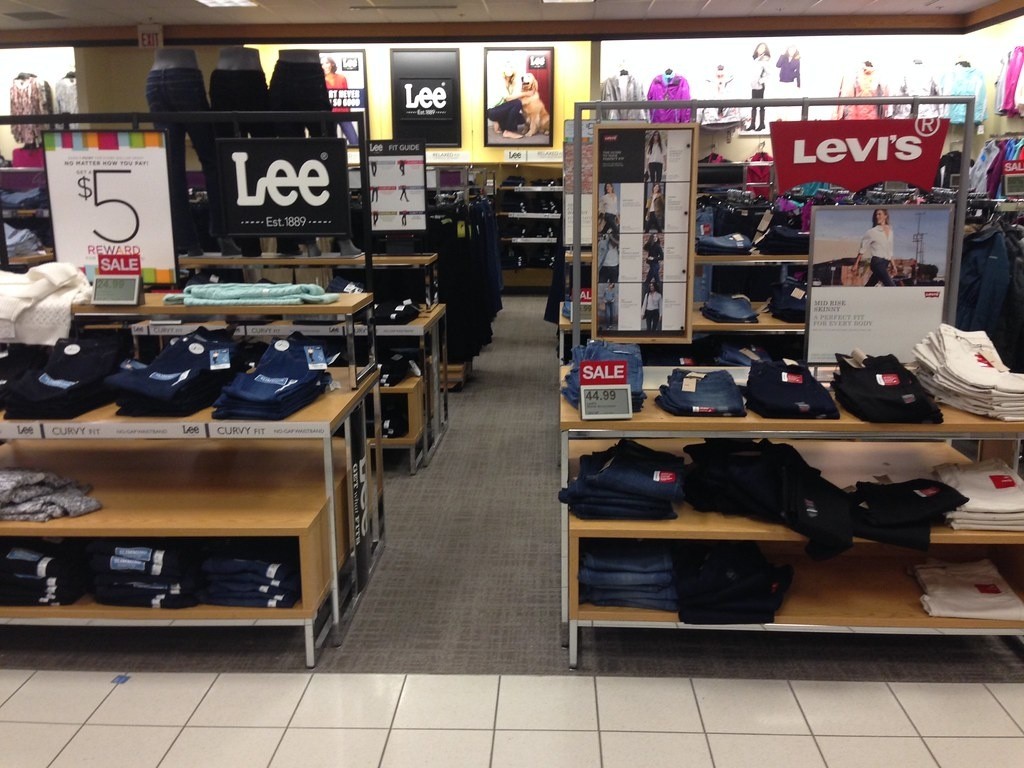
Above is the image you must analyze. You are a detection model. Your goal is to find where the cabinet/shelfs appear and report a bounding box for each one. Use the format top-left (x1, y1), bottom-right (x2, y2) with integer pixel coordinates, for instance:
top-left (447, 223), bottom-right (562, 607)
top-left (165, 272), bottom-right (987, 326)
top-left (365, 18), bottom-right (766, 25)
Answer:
top-left (0, 107), bottom-right (389, 670)
top-left (558, 232), bottom-right (811, 365)
top-left (7, 252), bottom-right (448, 475)
top-left (557, 98), bottom-right (1024, 671)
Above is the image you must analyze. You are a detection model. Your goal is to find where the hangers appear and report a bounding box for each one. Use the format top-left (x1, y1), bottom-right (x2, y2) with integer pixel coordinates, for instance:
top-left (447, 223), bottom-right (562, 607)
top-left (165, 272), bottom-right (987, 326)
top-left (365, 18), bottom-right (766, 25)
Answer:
top-left (864, 186), bottom-right (991, 205)
top-left (726, 189), bottom-right (756, 205)
top-left (788, 186), bottom-right (805, 199)
top-left (816, 189), bottom-right (850, 201)
top-left (616, 59), bottom-right (630, 78)
top-left (188, 187), bottom-right (209, 203)
top-left (452, 192), bottom-right (471, 210)
top-left (662, 61), bottom-right (676, 80)
top-left (989, 129), bottom-right (1022, 141)
top-left (435, 193), bottom-right (451, 209)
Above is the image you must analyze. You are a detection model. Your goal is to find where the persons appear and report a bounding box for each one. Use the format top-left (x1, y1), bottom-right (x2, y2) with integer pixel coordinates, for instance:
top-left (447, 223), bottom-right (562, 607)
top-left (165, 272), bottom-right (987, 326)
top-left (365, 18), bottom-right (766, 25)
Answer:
top-left (853, 209), bottom-right (900, 286)
top-left (486, 61), bottom-right (533, 138)
top-left (599, 283), bottom-right (618, 331)
top-left (645, 131), bottom-right (667, 181)
top-left (599, 184), bottom-right (619, 233)
top-left (317, 55), bottom-right (360, 145)
top-left (744, 42), bottom-right (802, 132)
top-left (642, 282), bottom-right (662, 332)
top-left (144, 46), bottom-right (242, 256)
top-left (208, 46), bottom-right (303, 258)
top-left (268, 47), bottom-right (363, 258)
top-left (642, 233), bottom-right (664, 282)
top-left (644, 183), bottom-right (665, 232)
top-left (598, 233), bottom-right (619, 282)
top-left (369, 160), bottom-right (410, 227)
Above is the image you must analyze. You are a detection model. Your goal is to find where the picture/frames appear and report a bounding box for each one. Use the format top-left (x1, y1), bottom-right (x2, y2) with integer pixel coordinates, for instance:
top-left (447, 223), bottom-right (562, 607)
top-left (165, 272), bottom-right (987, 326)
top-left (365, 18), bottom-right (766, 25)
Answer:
top-left (319, 48), bottom-right (370, 150)
top-left (390, 47), bottom-right (462, 148)
top-left (485, 47), bottom-right (553, 147)
